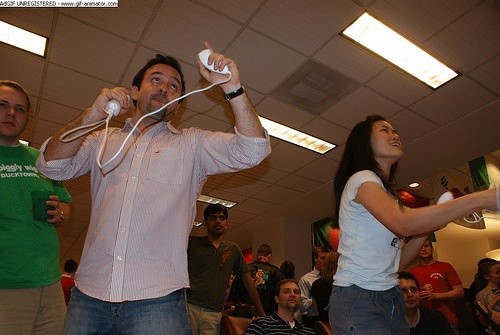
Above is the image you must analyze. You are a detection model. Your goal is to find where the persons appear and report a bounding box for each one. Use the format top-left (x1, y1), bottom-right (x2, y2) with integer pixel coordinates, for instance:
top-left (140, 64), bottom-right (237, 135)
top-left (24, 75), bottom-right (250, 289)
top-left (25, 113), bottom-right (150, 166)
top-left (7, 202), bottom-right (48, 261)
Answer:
top-left (1, 79), bottom-right (73, 334)
top-left (407, 235), bottom-right (465, 335)
top-left (298, 247), bottom-right (330, 325)
top-left (237, 244), bottom-right (284, 319)
top-left (328, 113), bottom-right (500, 335)
top-left (396, 271), bottom-right (453, 335)
top-left (475, 261), bottom-right (500, 330)
top-left (243, 279), bottom-right (316, 335)
top-left (309, 250), bottom-right (341, 322)
top-left (187, 204), bottom-right (269, 335)
top-left (470, 257), bottom-right (495, 304)
top-left (35, 40), bottom-right (272, 335)
top-left (60, 259), bottom-right (78, 306)
top-left (278, 260), bottom-right (295, 280)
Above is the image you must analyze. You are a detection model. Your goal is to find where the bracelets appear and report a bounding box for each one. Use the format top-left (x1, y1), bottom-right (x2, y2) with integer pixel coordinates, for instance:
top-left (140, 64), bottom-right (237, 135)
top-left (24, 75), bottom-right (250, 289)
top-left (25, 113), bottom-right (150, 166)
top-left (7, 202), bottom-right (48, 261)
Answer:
top-left (224, 85), bottom-right (246, 101)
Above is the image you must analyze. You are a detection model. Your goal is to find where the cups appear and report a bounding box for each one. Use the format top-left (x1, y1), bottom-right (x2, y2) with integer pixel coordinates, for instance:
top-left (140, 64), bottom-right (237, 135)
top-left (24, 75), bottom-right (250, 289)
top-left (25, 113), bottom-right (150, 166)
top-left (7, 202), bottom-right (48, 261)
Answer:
top-left (31, 190), bottom-right (55, 220)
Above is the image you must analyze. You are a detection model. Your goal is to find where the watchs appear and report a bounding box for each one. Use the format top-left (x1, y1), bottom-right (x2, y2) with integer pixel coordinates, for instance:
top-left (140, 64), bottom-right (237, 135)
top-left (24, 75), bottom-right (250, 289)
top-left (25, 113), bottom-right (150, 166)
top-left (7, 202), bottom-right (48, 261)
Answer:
top-left (59, 208), bottom-right (65, 226)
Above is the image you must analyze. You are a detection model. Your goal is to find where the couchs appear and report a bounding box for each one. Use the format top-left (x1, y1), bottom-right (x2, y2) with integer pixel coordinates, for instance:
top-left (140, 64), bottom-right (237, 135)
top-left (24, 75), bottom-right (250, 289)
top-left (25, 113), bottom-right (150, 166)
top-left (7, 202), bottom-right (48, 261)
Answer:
top-left (221, 313), bottom-right (254, 335)
top-left (316, 320), bottom-right (333, 335)
